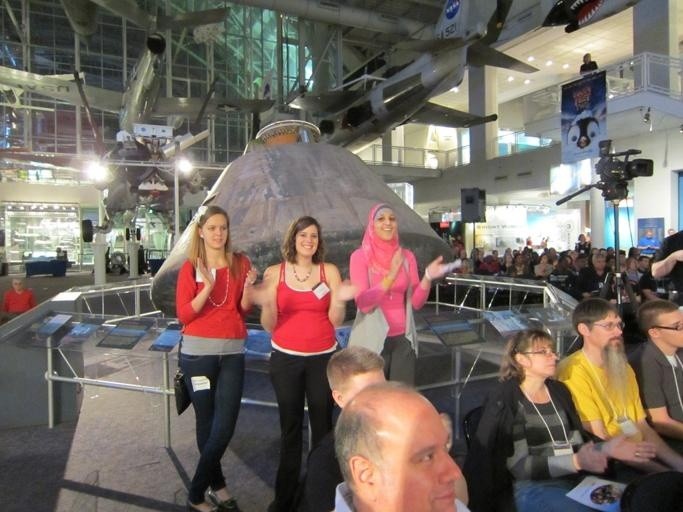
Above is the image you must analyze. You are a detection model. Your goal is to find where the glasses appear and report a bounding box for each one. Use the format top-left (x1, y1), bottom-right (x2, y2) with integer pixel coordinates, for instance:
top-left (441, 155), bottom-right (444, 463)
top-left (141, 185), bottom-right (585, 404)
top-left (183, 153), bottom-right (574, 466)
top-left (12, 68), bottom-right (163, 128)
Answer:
top-left (522, 348), bottom-right (560, 360)
top-left (595, 321), bottom-right (625, 330)
top-left (652, 324), bottom-right (683, 331)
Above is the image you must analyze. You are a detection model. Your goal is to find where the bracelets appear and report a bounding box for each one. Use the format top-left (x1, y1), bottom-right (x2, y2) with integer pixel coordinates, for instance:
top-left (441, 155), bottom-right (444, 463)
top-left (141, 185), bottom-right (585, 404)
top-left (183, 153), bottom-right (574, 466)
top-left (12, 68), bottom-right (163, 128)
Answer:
top-left (424, 268), bottom-right (433, 283)
top-left (382, 276), bottom-right (394, 288)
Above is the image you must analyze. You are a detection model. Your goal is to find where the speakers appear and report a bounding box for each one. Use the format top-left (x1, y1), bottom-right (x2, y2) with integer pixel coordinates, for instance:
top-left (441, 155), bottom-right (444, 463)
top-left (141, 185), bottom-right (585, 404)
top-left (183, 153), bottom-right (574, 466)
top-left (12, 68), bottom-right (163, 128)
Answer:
top-left (460, 186), bottom-right (486, 223)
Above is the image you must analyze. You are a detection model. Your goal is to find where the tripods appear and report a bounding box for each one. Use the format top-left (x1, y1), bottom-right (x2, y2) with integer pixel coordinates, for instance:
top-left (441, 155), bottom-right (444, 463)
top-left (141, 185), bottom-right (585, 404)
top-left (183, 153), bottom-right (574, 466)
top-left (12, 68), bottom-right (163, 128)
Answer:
top-left (593, 200), bottom-right (643, 320)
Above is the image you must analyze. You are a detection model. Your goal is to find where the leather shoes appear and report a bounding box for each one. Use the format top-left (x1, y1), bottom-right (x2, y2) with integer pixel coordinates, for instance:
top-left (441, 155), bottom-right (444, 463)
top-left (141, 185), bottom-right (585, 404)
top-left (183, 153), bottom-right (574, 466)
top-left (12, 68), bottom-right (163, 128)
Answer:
top-left (188, 497), bottom-right (222, 512)
top-left (208, 489), bottom-right (241, 512)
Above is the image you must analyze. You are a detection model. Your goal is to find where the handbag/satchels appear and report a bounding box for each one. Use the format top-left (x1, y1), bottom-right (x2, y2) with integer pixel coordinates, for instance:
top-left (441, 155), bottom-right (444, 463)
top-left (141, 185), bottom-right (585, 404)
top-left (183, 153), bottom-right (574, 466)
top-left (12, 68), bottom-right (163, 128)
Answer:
top-left (174, 370), bottom-right (192, 415)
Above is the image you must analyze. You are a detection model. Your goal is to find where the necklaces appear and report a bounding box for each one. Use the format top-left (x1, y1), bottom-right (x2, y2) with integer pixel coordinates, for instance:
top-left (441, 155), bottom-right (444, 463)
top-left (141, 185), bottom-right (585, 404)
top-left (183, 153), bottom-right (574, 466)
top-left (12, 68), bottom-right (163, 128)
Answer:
top-left (292, 261), bottom-right (313, 281)
top-left (207, 261), bottom-right (232, 308)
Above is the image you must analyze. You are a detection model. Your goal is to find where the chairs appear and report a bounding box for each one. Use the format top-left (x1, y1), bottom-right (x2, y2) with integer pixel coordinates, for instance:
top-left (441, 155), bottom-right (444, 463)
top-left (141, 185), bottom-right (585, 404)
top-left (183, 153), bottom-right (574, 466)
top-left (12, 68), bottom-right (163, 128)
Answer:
top-left (463, 402), bottom-right (522, 512)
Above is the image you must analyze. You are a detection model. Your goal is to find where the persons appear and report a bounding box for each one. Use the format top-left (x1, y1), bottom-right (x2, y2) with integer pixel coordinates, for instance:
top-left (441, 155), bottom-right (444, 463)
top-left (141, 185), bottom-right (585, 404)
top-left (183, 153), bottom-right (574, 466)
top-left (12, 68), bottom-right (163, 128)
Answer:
top-left (300, 345), bottom-right (470, 511)
top-left (349, 201), bottom-right (462, 380)
top-left (335, 378), bottom-right (472, 512)
top-left (260, 216), bottom-right (359, 509)
top-left (178, 201), bottom-right (259, 512)
top-left (455, 225), bottom-right (683, 511)
top-left (1, 277), bottom-right (37, 320)
top-left (580, 52), bottom-right (598, 76)
